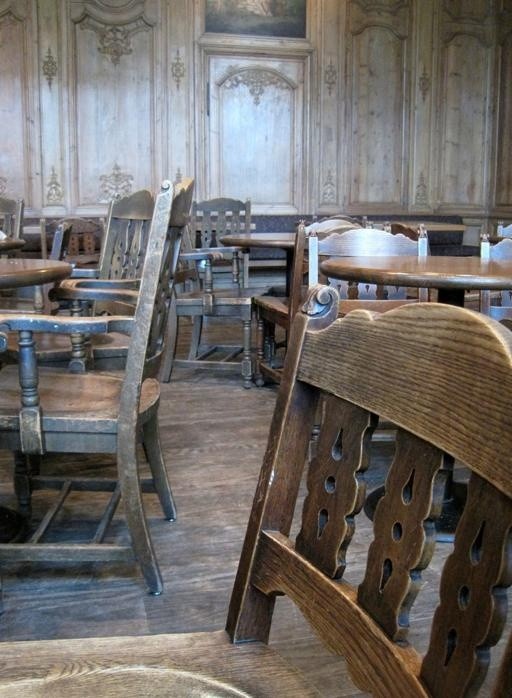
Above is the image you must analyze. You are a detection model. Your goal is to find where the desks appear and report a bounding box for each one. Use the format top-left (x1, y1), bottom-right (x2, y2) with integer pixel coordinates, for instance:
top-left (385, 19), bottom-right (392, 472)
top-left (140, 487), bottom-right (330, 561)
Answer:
top-left (320, 256), bottom-right (510, 541)
top-left (4, 257), bottom-right (73, 309)
top-left (220, 232), bottom-right (298, 297)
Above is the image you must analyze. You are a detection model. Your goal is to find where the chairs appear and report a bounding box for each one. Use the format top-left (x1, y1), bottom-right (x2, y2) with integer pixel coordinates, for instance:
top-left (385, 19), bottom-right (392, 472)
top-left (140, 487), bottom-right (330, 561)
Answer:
top-left (7, 190), bottom-right (160, 370)
top-left (180, 200), bottom-right (257, 389)
top-left (306, 233), bottom-right (432, 461)
top-left (1, 175), bottom-right (106, 270)
top-left (1, 285), bottom-right (512, 698)
top-left (253, 218), bottom-right (368, 388)
top-left (381, 219), bottom-right (508, 258)
top-left (480, 231), bottom-right (512, 326)
top-left (3, 177), bottom-right (197, 595)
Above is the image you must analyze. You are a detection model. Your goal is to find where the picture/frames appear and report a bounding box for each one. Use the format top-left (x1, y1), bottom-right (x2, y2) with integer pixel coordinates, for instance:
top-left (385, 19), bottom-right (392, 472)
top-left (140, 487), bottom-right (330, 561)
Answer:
top-left (194, 0), bottom-right (317, 50)
